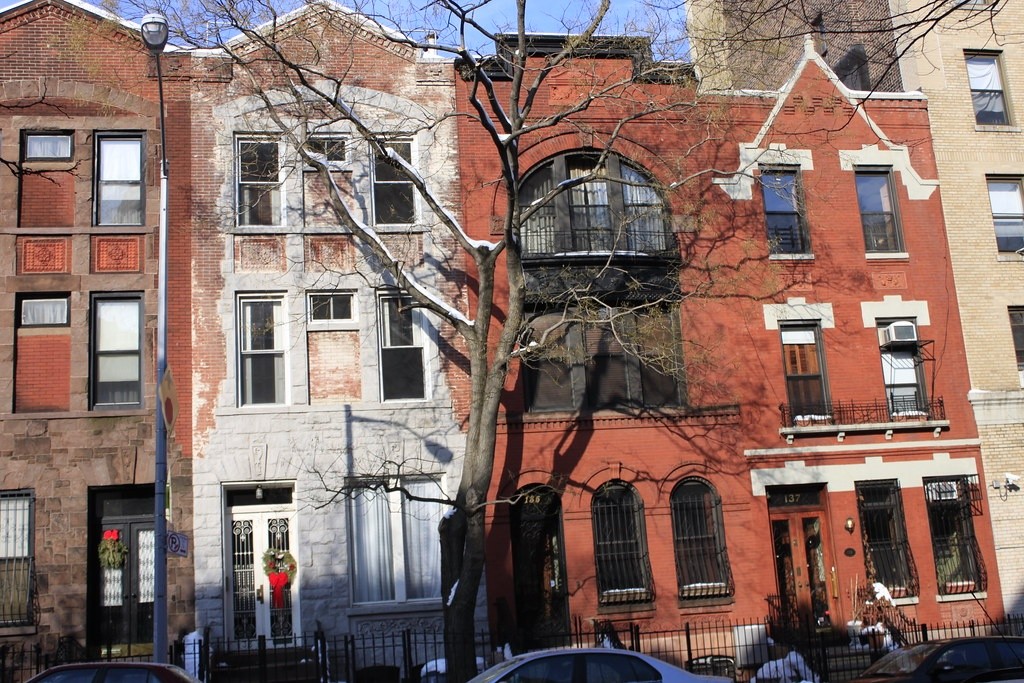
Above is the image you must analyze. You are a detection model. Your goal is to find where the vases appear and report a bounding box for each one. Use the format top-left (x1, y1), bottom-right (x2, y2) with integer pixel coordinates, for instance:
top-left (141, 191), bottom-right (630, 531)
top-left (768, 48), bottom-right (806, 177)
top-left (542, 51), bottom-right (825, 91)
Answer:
top-left (865, 631), bottom-right (887, 648)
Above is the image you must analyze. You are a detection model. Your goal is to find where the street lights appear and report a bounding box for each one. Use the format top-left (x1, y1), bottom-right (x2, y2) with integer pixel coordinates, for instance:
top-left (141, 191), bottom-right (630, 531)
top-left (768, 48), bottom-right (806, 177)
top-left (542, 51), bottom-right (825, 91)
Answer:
top-left (139, 10), bottom-right (170, 665)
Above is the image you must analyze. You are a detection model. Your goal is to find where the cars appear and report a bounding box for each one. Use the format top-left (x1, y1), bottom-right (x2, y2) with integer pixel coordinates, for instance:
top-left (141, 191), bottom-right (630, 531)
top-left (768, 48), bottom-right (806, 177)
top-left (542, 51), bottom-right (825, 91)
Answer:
top-left (28, 636), bottom-right (1024, 683)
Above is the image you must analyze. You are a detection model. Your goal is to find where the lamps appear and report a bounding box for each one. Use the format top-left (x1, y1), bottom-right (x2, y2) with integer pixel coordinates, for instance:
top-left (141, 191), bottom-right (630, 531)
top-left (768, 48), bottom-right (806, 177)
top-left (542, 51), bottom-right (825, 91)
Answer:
top-left (845, 517), bottom-right (854, 535)
top-left (1005, 470), bottom-right (1021, 488)
top-left (255, 483), bottom-right (263, 500)
